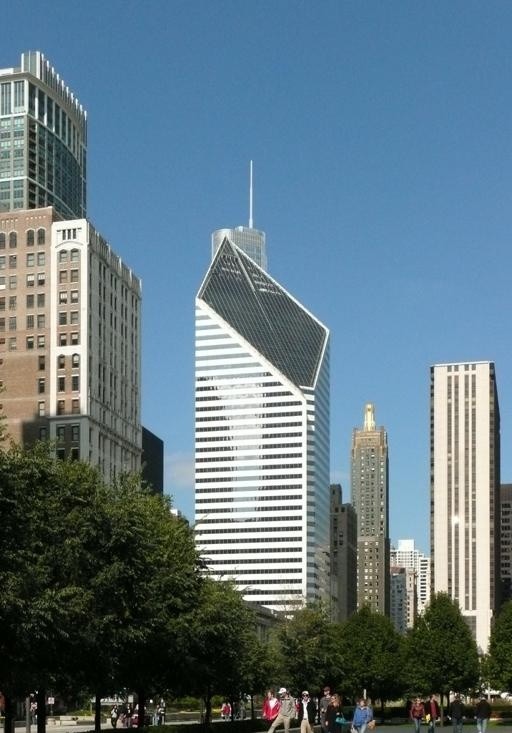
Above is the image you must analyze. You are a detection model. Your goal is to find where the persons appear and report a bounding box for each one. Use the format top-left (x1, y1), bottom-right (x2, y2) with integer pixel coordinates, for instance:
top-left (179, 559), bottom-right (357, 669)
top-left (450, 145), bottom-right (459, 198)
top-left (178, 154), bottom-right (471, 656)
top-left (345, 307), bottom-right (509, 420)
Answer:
top-left (110, 704), bottom-right (161, 728)
top-left (220, 685), bottom-right (492, 733)
top-left (30, 705), bottom-right (37, 724)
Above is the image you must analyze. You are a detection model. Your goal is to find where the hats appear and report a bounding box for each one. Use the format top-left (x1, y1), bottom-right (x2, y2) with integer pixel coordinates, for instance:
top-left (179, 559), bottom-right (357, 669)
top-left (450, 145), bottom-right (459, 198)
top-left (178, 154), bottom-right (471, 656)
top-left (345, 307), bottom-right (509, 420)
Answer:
top-left (322, 686), bottom-right (331, 691)
top-left (278, 687), bottom-right (287, 694)
top-left (301, 690), bottom-right (310, 695)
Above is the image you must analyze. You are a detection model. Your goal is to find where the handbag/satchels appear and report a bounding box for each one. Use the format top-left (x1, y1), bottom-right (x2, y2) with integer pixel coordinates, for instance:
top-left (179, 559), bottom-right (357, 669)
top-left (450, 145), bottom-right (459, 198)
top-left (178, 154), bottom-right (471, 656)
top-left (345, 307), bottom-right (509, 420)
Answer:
top-left (335, 716), bottom-right (348, 726)
top-left (366, 719), bottom-right (377, 731)
